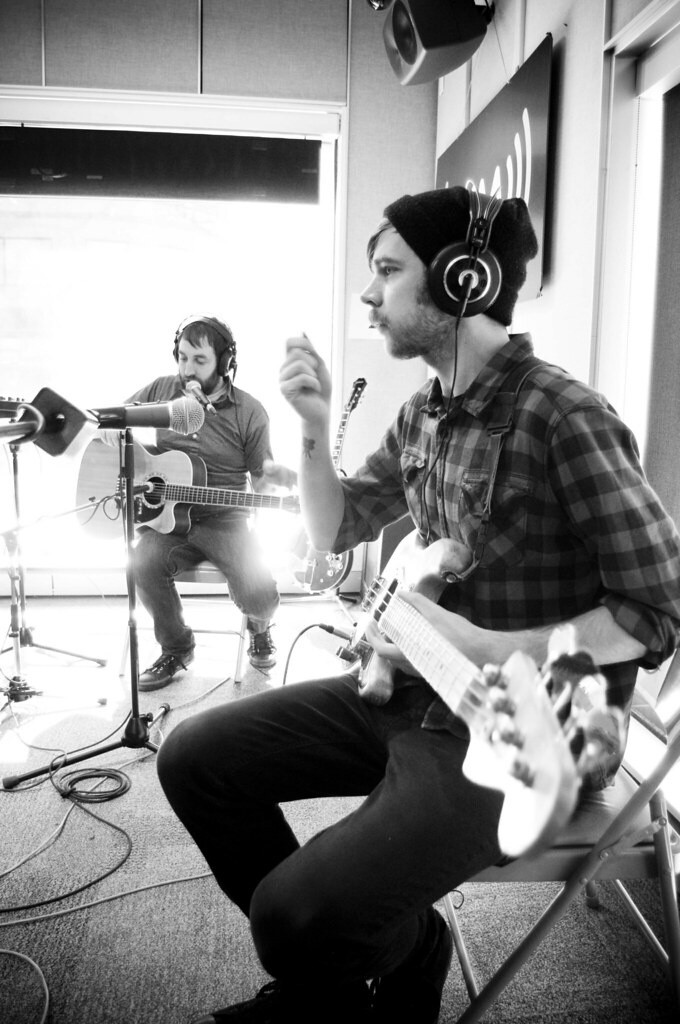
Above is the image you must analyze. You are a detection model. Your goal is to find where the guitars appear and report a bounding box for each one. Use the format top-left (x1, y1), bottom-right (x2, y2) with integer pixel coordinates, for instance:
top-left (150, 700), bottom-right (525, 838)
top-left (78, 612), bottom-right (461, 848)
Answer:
top-left (334, 529), bottom-right (587, 868)
top-left (75, 431), bottom-right (322, 536)
top-left (290, 376), bottom-right (368, 594)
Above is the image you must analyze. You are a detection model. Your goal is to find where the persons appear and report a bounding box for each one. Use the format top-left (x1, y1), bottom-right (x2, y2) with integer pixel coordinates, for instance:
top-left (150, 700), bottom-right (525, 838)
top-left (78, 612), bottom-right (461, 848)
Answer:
top-left (153, 181), bottom-right (680, 1024)
top-left (102, 320), bottom-right (288, 693)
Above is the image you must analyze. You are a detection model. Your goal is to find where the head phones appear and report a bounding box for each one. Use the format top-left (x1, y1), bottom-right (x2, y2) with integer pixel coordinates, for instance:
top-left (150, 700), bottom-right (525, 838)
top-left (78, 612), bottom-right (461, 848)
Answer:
top-left (428, 188), bottom-right (503, 318)
top-left (173, 315), bottom-right (237, 377)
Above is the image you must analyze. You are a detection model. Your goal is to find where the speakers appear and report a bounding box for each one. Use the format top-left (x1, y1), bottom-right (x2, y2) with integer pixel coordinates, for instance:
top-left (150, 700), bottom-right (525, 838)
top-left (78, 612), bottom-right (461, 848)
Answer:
top-left (382, 0), bottom-right (487, 85)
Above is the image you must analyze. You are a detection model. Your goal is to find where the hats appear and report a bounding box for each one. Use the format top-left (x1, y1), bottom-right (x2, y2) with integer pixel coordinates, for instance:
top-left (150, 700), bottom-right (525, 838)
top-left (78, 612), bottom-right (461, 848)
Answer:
top-left (383, 187), bottom-right (538, 325)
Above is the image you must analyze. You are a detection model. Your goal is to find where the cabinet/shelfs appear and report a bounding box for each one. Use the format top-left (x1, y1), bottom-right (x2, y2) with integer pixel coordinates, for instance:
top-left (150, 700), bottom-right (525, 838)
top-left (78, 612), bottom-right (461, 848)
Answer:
top-left (359, 514), bottom-right (416, 601)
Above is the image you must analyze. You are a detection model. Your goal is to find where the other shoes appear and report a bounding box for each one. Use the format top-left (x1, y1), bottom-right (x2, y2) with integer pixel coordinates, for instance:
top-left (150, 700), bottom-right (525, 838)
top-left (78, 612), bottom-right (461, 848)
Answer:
top-left (368, 908), bottom-right (452, 1024)
top-left (193, 973), bottom-right (372, 1024)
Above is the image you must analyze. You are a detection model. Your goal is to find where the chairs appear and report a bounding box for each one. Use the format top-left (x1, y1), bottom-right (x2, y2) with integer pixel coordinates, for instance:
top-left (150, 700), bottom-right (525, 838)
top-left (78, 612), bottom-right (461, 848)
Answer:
top-left (118, 558), bottom-right (249, 684)
top-left (441, 641), bottom-right (680, 1024)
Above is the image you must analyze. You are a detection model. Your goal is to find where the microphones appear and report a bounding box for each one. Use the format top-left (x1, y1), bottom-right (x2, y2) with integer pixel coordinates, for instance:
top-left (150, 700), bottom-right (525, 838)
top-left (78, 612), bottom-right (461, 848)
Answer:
top-left (85, 396), bottom-right (205, 435)
top-left (186, 379), bottom-right (217, 417)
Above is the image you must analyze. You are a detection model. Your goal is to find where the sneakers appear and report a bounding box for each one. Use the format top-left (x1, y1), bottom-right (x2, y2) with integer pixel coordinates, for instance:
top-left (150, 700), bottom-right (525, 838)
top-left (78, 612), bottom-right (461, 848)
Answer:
top-left (138, 649), bottom-right (194, 692)
top-left (247, 624), bottom-right (277, 667)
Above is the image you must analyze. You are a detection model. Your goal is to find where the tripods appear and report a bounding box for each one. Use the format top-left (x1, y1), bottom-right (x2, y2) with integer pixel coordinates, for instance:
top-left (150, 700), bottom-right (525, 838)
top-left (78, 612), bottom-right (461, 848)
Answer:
top-left (0, 424), bottom-right (169, 790)
